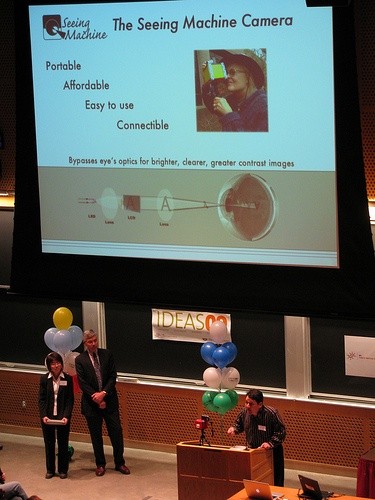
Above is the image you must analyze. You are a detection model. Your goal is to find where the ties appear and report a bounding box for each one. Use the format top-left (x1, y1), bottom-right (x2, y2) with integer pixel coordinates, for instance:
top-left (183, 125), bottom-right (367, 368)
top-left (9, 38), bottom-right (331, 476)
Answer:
top-left (92, 351), bottom-right (103, 392)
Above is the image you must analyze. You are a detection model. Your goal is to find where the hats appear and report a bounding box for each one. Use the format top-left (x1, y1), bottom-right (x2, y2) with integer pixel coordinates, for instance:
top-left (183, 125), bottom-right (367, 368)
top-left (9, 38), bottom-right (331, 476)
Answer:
top-left (208, 49), bottom-right (266, 90)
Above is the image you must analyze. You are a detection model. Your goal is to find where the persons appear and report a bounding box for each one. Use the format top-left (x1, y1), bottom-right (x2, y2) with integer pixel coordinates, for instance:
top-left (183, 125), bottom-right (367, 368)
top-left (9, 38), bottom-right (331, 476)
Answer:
top-left (38, 352), bottom-right (74, 479)
top-left (0, 468), bottom-right (29, 500)
top-left (228, 389), bottom-right (288, 487)
top-left (202, 50), bottom-right (268, 132)
top-left (74, 330), bottom-right (131, 476)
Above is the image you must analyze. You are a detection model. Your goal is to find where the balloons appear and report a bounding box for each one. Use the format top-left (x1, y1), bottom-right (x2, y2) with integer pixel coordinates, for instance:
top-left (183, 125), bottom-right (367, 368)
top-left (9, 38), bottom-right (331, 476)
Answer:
top-left (44, 307), bottom-right (83, 395)
top-left (201, 321), bottom-right (240, 414)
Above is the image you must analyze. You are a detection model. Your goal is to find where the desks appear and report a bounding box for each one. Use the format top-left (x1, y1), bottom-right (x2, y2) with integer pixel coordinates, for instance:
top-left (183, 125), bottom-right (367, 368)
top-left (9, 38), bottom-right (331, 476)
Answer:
top-left (226, 446), bottom-right (375, 500)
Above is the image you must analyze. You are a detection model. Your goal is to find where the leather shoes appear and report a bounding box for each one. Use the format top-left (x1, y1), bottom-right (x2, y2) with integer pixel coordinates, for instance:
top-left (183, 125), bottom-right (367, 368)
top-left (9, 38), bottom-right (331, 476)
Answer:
top-left (95, 465), bottom-right (105, 476)
top-left (115, 464), bottom-right (131, 474)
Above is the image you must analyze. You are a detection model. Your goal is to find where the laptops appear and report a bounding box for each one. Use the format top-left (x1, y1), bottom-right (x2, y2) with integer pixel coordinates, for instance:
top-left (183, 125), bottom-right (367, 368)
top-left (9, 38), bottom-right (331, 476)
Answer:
top-left (298, 474), bottom-right (327, 498)
top-left (243, 479), bottom-right (284, 500)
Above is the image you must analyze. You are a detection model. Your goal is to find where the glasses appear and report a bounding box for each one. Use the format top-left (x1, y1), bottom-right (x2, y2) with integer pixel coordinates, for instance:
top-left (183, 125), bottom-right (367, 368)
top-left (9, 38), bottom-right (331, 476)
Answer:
top-left (226, 67), bottom-right (249, 76)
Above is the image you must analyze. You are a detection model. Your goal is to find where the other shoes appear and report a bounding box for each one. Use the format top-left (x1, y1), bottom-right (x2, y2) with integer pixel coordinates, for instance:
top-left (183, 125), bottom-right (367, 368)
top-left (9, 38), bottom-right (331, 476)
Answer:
top-left (60, 473), bottom-right (67, 479)
top-left (46, 473), bottom-right (53, 479)
